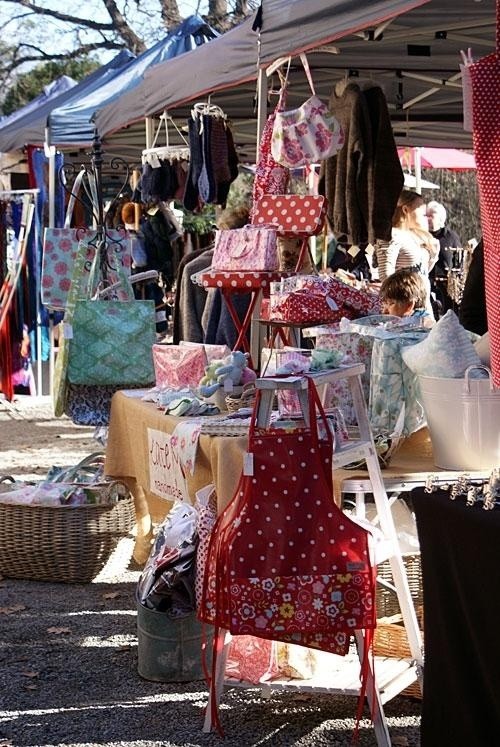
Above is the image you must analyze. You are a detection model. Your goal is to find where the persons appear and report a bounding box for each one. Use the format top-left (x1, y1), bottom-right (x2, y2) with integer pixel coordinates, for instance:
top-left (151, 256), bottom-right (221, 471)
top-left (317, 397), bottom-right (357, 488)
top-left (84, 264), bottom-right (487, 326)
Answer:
top-left (206, 204), bottom-right (252, 352)
top-left (380, 268), bottom-right (435, 326)
top-left (389, 190), bottom-right (442, 329)
top-left (426, 200), bottom-right (462, 320)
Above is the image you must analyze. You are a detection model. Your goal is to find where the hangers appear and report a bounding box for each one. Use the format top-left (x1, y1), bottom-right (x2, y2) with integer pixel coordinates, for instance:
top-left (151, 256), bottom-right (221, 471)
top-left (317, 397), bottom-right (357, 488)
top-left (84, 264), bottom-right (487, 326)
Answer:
top-left (333, 68), bottom-right (380, 97)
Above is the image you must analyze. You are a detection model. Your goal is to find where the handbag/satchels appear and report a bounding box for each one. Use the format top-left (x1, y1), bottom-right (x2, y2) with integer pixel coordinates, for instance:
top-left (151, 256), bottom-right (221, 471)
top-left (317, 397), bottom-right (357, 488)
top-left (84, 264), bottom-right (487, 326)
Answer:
top-left (212, 98), bottom-right (346, 271)
top-left (41, 228), bottom-right (156, 428)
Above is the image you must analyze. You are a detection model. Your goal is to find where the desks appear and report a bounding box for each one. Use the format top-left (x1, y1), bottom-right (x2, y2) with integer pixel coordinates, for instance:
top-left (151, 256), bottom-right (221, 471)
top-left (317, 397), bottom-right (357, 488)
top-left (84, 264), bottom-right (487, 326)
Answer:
top-left (112, 392), bottom-right (500, 683)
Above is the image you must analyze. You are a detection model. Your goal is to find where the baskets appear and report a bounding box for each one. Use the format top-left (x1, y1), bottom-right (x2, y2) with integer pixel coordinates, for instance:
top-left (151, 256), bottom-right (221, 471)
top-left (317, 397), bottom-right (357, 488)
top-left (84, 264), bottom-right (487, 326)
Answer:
top-left (373, 617), bottom-right (424, 700)
top-left (0, 476), bottom-right (136, 584)
top-left (376, 553), bottom-right (424, 619)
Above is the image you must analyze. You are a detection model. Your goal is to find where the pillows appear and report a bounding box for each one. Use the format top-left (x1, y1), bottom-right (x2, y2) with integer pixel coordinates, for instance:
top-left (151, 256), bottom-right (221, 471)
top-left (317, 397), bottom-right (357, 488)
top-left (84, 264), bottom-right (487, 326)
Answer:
top-left (398, 309), bottom-right (488, 379)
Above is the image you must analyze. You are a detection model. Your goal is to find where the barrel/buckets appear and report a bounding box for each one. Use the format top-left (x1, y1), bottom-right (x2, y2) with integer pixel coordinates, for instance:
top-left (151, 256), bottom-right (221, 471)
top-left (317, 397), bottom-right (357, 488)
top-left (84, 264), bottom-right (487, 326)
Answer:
top-left (133, 585), bottom-right (214, 683)
top-left (416, 362), bottom-right (500, 472)
